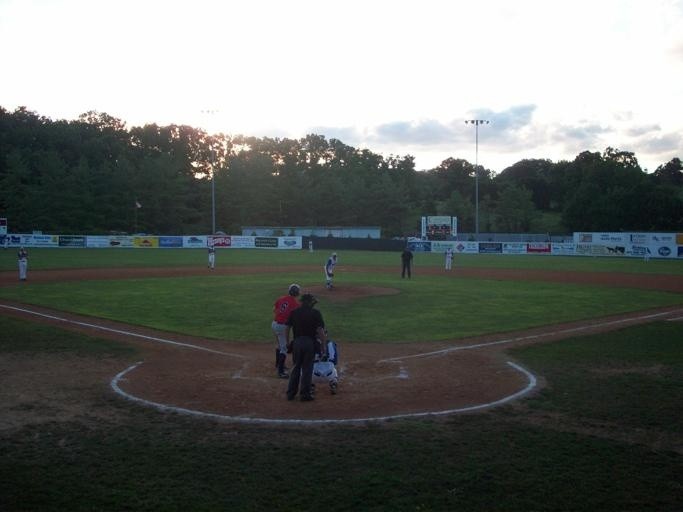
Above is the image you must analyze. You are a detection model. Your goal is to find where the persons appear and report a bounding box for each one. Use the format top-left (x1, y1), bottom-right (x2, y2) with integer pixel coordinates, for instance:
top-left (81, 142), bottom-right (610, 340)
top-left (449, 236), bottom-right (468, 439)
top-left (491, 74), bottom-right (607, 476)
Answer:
top-left (324, 252), bottom-right (337, 289)
top-left (443, 248), bottom-right (454, 272)
top-left (208, 244), bottom-right (215, 269)
top-left (401, 247), bottom-right (413, 278)
top-left (270, 283), bottom-right (340, 402)
top-left (17, 245), bottom-right (30, 280)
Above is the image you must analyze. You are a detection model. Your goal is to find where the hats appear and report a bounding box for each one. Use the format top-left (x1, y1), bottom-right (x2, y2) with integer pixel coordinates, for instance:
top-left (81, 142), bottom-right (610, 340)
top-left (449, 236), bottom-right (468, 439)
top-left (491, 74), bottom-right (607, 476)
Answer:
top-left (300, 294), bottom-right (319, 302)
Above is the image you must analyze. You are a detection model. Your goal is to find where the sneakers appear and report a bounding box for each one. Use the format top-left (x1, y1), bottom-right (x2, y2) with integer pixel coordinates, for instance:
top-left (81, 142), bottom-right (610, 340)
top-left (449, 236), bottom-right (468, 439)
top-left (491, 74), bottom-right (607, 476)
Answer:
top-left (331, 384), bottom-right (337, 394)
top-left (286, 384), bottom-right (316, 401)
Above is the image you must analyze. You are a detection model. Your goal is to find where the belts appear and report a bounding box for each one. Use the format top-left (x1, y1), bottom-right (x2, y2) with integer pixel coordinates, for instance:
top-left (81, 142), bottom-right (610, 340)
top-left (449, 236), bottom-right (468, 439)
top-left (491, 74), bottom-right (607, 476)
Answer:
top-left (275, 319), bottom-right (289, 325)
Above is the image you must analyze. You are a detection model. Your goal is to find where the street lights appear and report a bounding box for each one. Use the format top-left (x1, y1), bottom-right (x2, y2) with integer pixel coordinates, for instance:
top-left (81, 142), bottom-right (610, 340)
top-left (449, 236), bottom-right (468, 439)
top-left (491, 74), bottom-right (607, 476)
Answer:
top-left (463, 119), bottom-right (490, 233)
top-left (195, 106), bottom-right (225, 235)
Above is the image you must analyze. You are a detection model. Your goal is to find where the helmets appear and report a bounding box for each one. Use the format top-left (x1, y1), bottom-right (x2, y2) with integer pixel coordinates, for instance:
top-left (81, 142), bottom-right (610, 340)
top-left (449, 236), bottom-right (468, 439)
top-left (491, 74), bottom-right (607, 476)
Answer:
top-left (288, 284), bottom-right (300, 297)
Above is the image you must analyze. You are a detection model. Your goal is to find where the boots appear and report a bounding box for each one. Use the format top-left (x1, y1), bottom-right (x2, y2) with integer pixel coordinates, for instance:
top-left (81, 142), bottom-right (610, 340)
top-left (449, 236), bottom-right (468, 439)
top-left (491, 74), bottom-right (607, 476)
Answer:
top-left (274, 349), bottom-right (289, 378)
